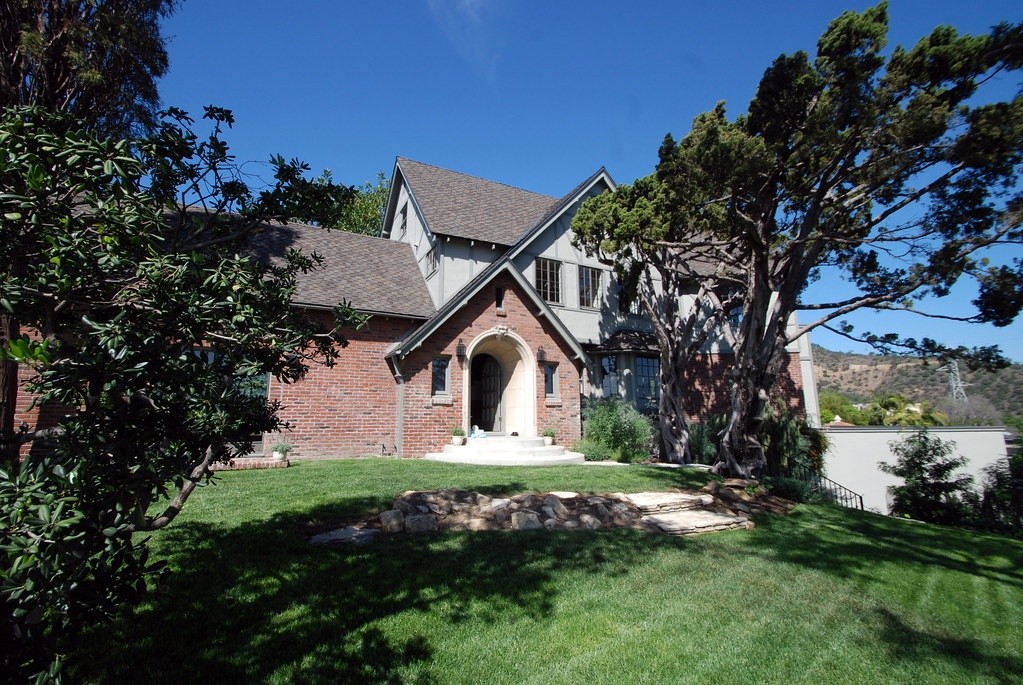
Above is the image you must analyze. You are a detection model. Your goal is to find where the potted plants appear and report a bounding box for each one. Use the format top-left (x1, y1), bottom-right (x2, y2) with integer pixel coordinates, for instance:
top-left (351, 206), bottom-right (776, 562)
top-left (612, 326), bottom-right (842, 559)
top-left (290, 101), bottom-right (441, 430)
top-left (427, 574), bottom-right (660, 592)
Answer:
top-left (269, 439), bottom-right (292, 462)
top-left (450, 427), bottom-right (465, 446)
top-left (541, 428), bottom-right (556, 446)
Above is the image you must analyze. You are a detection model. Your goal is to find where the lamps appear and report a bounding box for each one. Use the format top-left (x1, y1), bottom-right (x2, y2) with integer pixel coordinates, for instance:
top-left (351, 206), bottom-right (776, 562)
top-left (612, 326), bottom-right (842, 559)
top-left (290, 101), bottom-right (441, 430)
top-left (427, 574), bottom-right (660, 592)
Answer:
top-left (415, 241), bottom-right (419, 247)
top-left (456, 337), bottom-right (466, 363)
top-left (537, 344), bottom-right (547, 366)
top-left (432, 235), bottom-right (495, 250)
